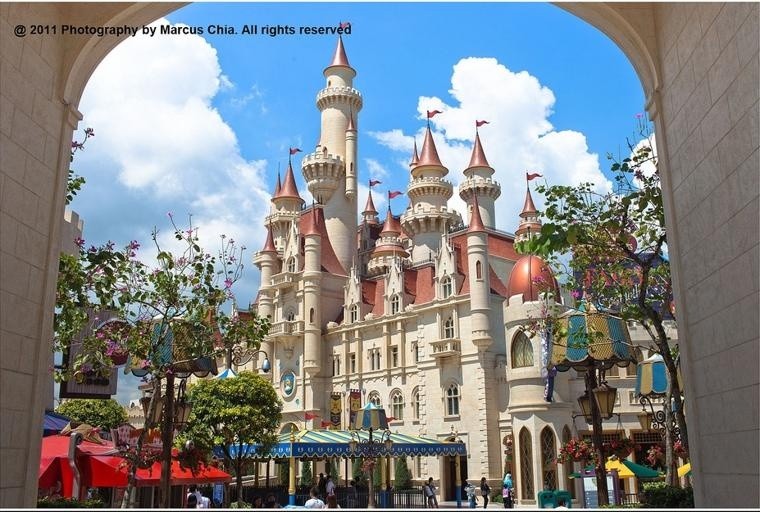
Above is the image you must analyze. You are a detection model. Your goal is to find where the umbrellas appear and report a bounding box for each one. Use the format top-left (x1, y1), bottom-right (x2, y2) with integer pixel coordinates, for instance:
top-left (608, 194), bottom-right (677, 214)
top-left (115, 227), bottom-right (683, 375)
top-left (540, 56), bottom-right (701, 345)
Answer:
top-left (567, 456), bottom-right (666, 479)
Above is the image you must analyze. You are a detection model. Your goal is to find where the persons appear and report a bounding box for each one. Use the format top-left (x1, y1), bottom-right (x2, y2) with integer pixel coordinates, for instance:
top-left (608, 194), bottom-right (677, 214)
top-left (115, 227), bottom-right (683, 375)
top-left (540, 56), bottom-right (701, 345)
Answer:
top-left (422, 481), bottom-right (436, 508)
top-left (503, 470), bottom-right (513, 489)
top-left (186, 472), bottom-right (368, 508)
top-left (463, 480), bottom-right (479, 506)
top-left (502, 483), bottom-right (512, 508)
top-left (428, 477), bottom-right (440, 508)
top-left (480, 477), bottom-right (491, 508)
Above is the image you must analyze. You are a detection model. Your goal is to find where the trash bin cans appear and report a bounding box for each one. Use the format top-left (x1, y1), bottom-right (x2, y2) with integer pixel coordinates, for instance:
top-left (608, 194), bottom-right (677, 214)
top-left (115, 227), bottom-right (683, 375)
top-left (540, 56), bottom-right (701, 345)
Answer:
top-left (380, 492), bottom-right (393, 508)
top-left (538, 491), bottom-right (571, 508)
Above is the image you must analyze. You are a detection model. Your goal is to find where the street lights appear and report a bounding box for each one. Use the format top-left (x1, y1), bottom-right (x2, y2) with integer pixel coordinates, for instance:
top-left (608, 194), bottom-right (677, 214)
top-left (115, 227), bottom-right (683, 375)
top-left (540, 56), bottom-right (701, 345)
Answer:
top-left (126, 314), bottom-right (217, 511)
top-left (191, 319), bottom-right (272, 509)
top-left (632, 345), bottom-right (688, 510)
top-left (547, 294), bottom-right (643, 511)
top-left (348, 400), bottom-right (395, 511)
